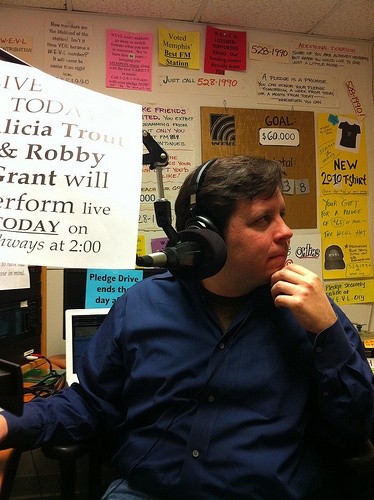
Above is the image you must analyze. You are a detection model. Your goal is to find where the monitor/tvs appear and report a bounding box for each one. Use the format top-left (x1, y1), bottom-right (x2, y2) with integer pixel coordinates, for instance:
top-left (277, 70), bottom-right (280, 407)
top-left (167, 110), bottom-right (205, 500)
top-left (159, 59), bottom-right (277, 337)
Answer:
top-left (65, 308), bottom-right (113, 387)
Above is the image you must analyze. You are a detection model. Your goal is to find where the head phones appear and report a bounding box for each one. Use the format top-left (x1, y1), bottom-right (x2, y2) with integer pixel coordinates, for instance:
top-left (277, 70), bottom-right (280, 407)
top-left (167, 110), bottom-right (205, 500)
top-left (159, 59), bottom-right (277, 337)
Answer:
top-left (185, 158), bottom-right (217, 231)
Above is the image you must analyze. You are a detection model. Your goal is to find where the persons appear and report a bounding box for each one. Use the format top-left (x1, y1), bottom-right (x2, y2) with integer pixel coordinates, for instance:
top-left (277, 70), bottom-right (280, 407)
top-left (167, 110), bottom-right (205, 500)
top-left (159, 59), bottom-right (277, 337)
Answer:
top-left (0, 155), bottom-right (374, 500)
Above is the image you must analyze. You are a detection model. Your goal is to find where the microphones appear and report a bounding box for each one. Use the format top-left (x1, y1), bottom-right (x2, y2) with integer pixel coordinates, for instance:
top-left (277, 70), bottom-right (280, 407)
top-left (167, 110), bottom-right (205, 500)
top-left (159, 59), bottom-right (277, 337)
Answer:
top-left (137, 229), bottom-right (227, 281)
